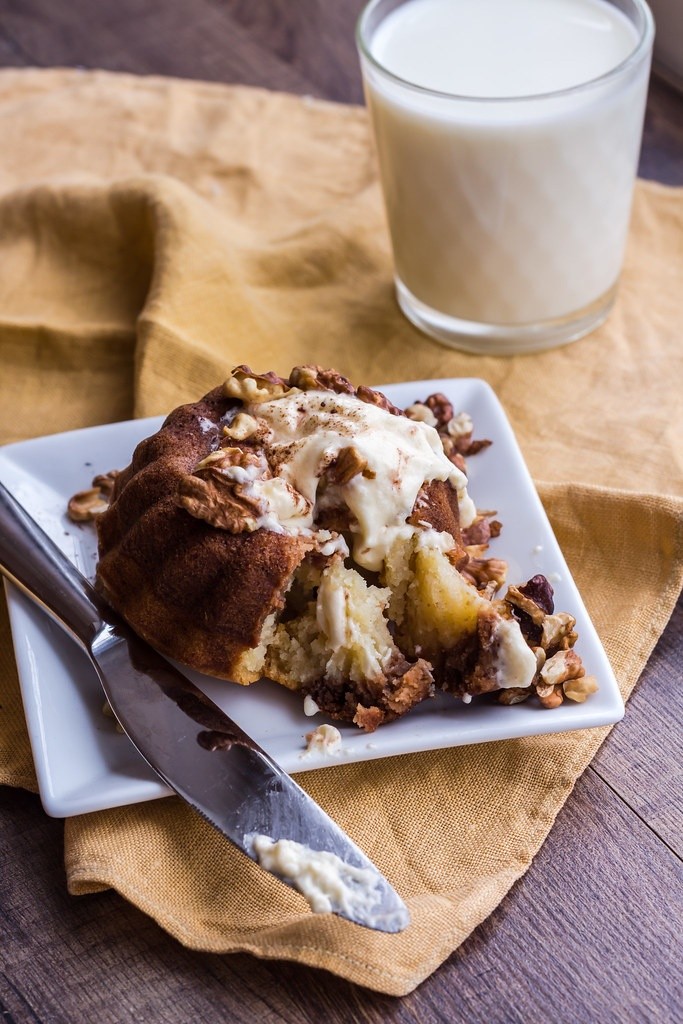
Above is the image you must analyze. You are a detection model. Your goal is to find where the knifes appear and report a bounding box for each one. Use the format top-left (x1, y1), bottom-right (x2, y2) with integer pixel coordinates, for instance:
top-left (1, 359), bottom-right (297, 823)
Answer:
top-left (2, 475), bottom-right (413, 942)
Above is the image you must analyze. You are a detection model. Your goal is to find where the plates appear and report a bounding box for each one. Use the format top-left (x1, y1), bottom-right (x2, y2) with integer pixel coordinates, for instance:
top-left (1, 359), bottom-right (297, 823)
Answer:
top-left (2, 370), bottom-right (625, 824)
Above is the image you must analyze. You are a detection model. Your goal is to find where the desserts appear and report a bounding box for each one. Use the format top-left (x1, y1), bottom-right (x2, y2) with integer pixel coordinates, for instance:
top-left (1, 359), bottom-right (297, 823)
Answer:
top-left (62, 361), bottom-right (598, 748)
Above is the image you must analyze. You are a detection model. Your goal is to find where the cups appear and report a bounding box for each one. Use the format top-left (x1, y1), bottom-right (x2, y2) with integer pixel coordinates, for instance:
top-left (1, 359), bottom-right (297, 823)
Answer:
top-left (352, 1), bottom-right (655, 362)
